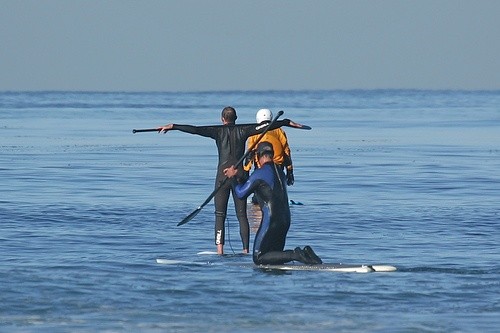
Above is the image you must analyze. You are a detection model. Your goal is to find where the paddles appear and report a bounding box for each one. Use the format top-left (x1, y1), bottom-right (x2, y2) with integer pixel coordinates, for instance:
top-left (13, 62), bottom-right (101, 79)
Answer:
top-left (130, 119), bottom-right (313, 134)
top-left (174, 110), bottom-right (286, 228)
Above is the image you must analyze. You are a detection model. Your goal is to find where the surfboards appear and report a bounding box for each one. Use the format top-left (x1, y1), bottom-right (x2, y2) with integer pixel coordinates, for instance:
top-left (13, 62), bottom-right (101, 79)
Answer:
top-left (207, 201), bottom-right (302, 206)
top-left (198, 249), bottom-right (253, 256)
top-left (158, 258), bottom-right (394, 272)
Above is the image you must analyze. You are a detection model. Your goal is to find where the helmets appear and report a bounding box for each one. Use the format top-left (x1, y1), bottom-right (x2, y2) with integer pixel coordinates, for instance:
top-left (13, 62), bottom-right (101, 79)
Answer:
top-left (256, 109), bottom-right (273, 124)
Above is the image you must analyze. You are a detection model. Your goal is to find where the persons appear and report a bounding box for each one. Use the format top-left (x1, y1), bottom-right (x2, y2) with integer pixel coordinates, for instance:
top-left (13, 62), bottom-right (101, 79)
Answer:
top-left (223, 141), bottom-right (322, 265)
top-left (243, 109), bottom-right (294, 204)
top-left (156, 106), bottom-right (312, 255)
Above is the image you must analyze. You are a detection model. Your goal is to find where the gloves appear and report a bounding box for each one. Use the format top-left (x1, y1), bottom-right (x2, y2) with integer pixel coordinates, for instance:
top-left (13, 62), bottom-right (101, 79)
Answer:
top-left (243, 170), bottom-right (249, 183)
top-left (286, 169), bottom-right (294, 186)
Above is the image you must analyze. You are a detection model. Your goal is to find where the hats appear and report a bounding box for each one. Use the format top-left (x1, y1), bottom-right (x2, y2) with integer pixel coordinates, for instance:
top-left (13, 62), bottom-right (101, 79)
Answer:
top-left (256, 142), bottom-right (273, 154)
